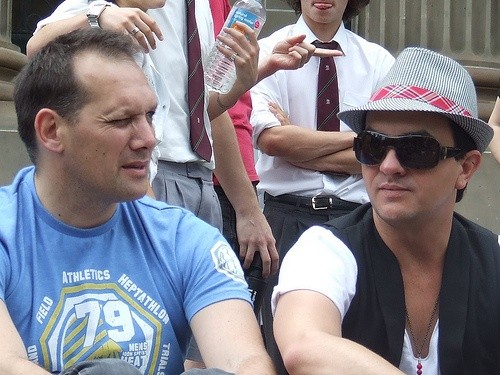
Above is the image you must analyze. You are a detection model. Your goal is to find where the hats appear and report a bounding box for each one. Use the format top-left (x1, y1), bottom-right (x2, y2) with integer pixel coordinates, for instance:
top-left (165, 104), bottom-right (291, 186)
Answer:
top-left (337, 48), bottom-right (494, 154)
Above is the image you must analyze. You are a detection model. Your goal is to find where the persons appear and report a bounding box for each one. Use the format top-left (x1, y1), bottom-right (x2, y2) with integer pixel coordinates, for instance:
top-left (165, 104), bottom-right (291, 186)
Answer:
top-left (269, 48), bottom-right (500, 375)
top-left (242, 0), bottom-right (395, 375)
top-left (209, 2), bottom-right (269, 314)
top-left (0, 25), bottom-right (278, 375)
top-left (485, 96), bottom-right (500, 161)
top-left (25, 0), bottom-right (261, 272)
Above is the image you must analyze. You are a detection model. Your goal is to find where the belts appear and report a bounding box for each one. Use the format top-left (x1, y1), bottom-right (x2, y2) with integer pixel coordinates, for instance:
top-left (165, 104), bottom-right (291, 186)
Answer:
top-left (266, 195), bottom-right (362, 209)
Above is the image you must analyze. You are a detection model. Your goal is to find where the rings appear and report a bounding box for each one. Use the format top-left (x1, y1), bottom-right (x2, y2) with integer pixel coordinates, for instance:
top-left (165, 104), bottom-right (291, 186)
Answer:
top-left (131, 27), bottom-right (139, 35)
top-left (228, 52), bottom-right (238, 62)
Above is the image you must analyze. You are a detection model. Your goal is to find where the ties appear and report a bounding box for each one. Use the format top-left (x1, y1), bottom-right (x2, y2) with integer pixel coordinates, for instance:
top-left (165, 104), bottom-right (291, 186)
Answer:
top-left (311, 40), bottom-right (351, 175)
top-left (186, 0), bottom-right (212, 163)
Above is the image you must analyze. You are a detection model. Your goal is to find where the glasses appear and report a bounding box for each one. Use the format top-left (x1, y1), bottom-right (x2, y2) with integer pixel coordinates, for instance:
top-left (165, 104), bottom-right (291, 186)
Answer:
top-left (353, 131), bottom-right (462, 165)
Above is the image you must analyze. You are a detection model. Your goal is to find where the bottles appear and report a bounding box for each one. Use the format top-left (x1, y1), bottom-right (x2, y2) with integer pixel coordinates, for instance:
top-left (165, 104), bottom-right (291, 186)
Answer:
top-left (204, 0), bottom-right (266, 94)
top-left (245, 251), bottom-right (266, 313)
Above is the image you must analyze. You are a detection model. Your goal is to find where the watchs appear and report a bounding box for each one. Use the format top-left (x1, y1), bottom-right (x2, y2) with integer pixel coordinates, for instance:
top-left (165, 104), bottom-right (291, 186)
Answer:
top-left (85, 1), bottom-right (112, 31)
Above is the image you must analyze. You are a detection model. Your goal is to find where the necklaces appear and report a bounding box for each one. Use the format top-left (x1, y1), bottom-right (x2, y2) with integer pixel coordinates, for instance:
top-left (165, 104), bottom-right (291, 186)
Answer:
top-left (406, 292), bottom-right (438, 375)
